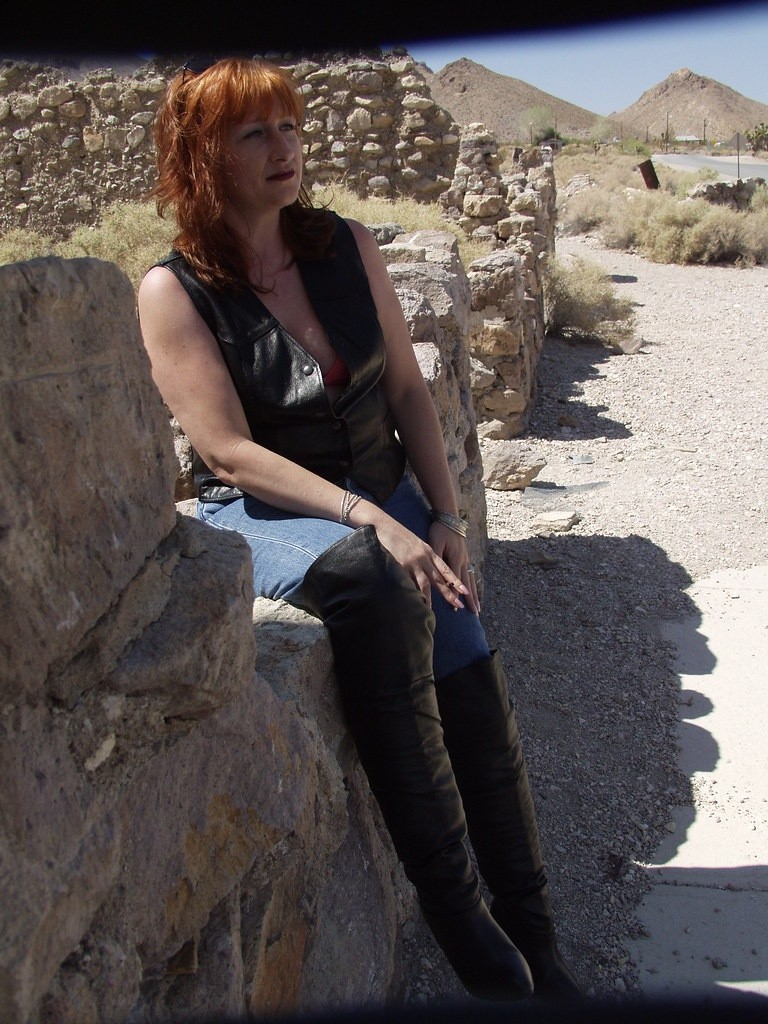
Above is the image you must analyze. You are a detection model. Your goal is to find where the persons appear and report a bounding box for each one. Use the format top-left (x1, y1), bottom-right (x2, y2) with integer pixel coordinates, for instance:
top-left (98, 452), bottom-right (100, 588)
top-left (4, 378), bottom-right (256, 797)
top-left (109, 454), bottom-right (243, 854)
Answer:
top-left (134, 58), bottom-right (583, 1003)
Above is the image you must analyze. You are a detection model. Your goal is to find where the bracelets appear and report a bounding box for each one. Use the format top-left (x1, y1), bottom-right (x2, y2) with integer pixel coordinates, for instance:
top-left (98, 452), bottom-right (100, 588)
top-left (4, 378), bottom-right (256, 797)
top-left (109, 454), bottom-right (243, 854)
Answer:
top-left (430, 508), bottom-right (470, 538)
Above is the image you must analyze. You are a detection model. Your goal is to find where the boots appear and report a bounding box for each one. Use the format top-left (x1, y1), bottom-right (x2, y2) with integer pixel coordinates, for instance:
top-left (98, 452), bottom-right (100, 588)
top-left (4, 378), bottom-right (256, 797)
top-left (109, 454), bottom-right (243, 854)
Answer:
top-left (436, 651), bottom-right (577, 996)
top-left (284, 522), bottom-right (538, 1001)
